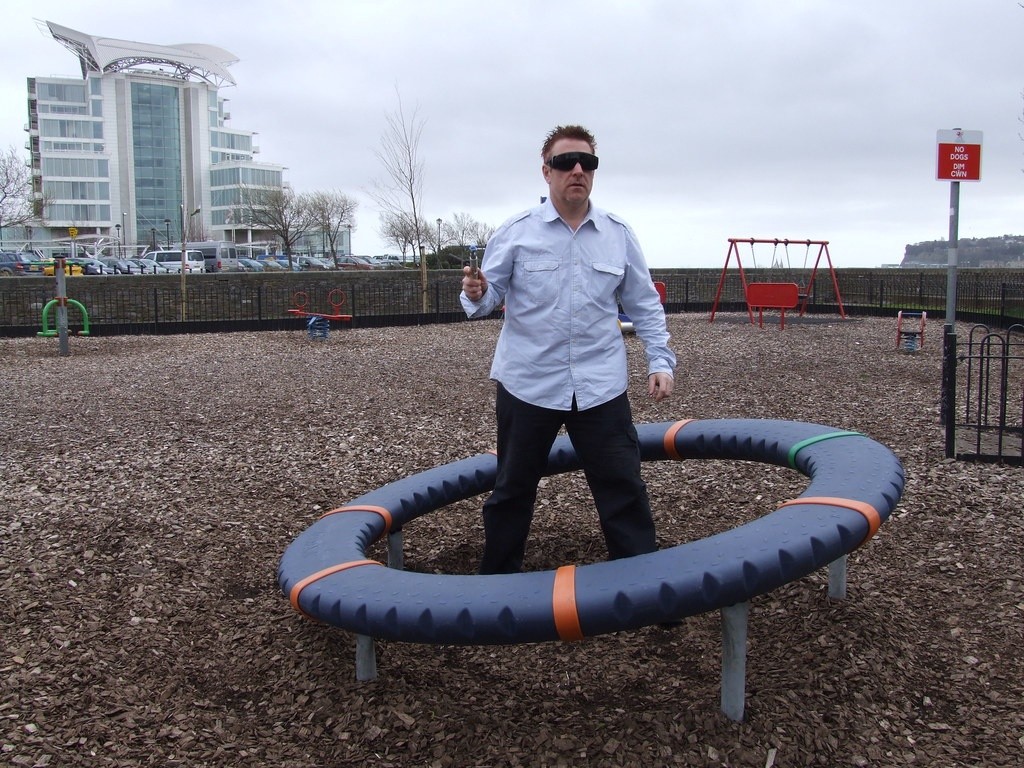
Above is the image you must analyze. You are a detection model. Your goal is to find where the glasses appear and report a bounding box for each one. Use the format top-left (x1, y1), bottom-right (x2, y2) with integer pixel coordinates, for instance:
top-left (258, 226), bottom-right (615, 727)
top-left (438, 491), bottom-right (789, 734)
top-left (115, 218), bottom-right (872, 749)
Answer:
top-left (547, 152), bottom-right (599, 171)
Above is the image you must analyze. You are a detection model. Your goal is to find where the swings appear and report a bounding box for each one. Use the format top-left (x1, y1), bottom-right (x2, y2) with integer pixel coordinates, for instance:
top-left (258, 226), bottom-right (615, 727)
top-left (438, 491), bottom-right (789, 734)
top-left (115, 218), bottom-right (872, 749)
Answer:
top-left (785, 246), bottom-right (808, 296)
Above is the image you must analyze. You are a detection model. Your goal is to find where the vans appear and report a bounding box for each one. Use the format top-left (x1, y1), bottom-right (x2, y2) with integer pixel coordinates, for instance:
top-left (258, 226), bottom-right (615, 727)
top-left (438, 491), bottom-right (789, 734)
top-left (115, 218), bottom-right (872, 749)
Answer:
top-left (94, 241), bottom-right (283, 273)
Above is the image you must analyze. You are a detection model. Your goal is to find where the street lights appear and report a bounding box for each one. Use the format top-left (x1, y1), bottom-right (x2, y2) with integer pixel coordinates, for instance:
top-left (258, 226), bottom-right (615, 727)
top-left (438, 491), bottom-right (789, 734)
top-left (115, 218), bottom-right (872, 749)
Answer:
top-left (436, 218), bottom-right (442, 270)
top-left (123, 213), bottom-right (127, 259)
top-left (164, 218), bottom-right (172, 250)
top-left (151, 227), bottom-right (156, 251)
top-left (347, 224), bottom-right (352, 256)
top-left (115, 224), bottom-right (122, 260)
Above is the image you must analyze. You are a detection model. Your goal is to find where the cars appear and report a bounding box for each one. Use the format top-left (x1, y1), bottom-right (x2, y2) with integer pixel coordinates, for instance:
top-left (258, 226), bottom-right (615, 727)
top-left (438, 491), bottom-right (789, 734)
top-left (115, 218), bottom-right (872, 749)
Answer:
top-left (238, 256), bottom-right (414, 272)
top-left (0, 250), bottom-right (169, 276)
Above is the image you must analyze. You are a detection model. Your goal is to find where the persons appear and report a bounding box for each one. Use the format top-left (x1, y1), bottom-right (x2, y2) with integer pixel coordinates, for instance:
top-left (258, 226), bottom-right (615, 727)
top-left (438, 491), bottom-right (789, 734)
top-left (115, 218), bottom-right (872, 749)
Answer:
top-left (460, 124), bottom-right (677, 575)
